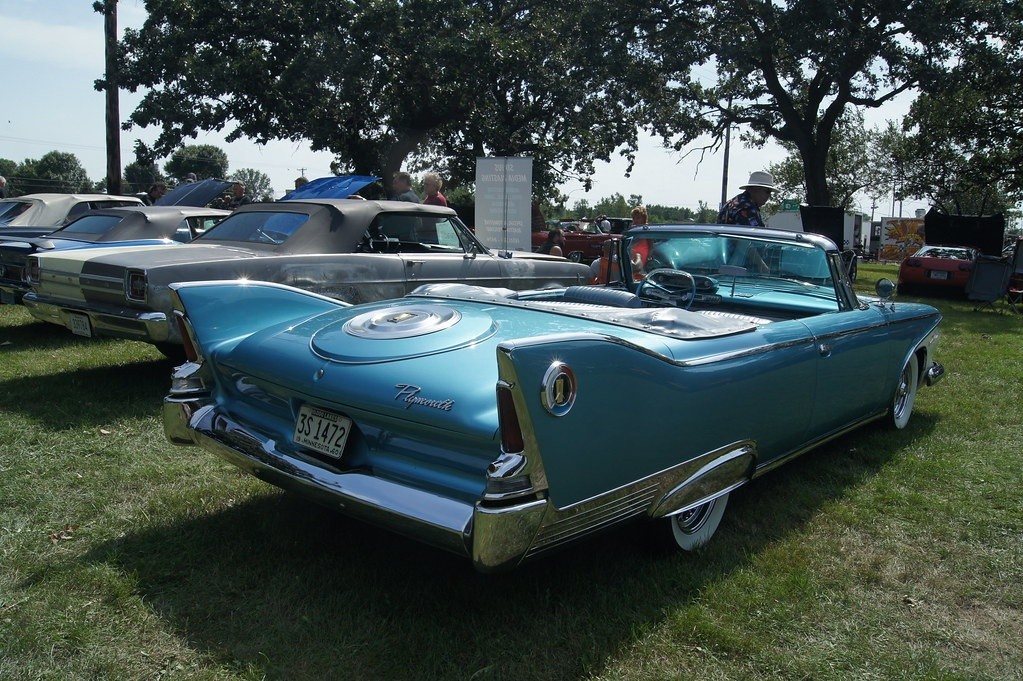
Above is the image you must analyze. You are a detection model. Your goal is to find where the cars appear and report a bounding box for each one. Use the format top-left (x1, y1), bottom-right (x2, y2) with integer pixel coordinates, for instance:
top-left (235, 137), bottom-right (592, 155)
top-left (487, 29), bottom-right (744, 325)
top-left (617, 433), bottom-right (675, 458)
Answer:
top-left (161, 221), bottom-right (946, 577)
top-left (21, 196), bottom-right (596, 359)
top-left (1, 179), bottom-right (234, 237)
top-left (531, 222), bottom-right (623, 261)
top-left (779, 247), bottom-right (857, 285)
top-left (0, 176), bottom-right (382, 294)
top-left (898, 246), bottom-right (980, 298)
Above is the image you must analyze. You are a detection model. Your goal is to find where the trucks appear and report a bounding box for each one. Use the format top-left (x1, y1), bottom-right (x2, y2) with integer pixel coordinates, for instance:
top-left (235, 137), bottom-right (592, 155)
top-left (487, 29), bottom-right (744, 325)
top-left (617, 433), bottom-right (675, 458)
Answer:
top-left (764, 209), bottom-right (863, 256)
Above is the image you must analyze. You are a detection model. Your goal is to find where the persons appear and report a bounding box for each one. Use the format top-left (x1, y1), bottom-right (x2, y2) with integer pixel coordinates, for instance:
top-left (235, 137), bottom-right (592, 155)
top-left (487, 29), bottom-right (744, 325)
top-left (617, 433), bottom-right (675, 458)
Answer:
top-left (142, 169), bottom-right (310, 213)
top-left (714, 169), bottom-right (782, 274)
top-left (588, 237), bottom-right (626, 287)
top-left (385, 173), bottom-right (423, 244)
top-left (534, 228), bottom-right (568, 257)
top-left (626, 207), bottom-right (653, 283)
top-left (0, 173), bottom-right (7, 199)
top-left (600, 214), bottom-right (612, 235)
top-left (416, 172), bottom-right (448, 247)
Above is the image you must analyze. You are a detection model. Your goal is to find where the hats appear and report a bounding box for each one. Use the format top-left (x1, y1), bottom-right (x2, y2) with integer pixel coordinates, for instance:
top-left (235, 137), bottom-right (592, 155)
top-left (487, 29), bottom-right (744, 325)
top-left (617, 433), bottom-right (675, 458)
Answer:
top-left (739, 171), bottom-right (781, 194)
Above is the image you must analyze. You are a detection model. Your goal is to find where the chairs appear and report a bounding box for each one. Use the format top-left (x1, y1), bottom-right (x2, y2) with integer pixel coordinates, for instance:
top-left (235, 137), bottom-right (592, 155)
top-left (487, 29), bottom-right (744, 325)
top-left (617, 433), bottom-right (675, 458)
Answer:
top-left (562, 285), bottom-right (641, 308)
